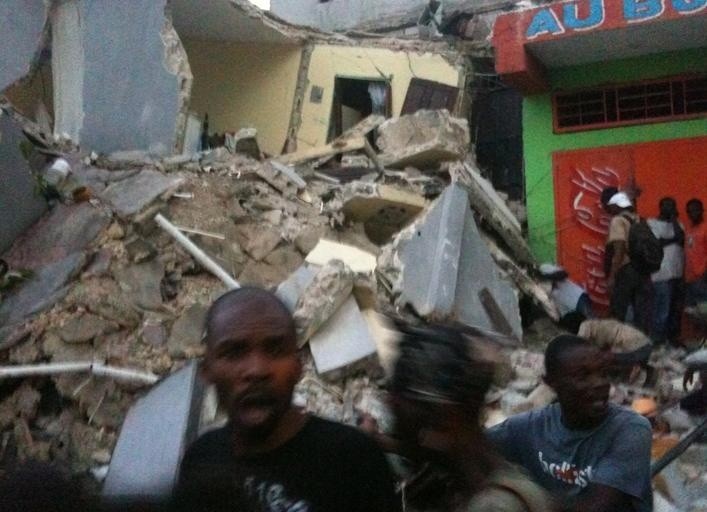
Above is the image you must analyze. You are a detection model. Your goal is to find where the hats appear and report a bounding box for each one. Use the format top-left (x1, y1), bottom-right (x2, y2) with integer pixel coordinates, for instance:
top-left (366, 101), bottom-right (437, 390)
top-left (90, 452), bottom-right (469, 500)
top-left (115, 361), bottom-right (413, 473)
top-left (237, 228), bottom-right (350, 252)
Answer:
top-left (607, 190), bottom-right (634, 208)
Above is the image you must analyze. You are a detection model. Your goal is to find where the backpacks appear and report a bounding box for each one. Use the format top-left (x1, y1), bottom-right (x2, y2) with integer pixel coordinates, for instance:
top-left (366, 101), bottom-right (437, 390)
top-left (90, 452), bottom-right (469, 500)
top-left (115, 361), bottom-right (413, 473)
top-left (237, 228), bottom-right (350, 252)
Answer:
top-left (621, 214), bottom-right (663, 274)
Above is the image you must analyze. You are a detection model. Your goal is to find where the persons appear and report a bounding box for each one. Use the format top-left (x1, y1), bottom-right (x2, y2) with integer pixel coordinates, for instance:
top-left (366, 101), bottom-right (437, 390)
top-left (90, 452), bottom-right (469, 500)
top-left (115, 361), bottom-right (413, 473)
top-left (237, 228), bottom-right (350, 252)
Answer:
top-left (600, 188), bottom-right (707, 350)
top-left (358, 335), bottom-right (651, 511)
top-left (679, 302), bottom-right (707, 441)
top-left (393, 320), bottom-right (559, 512)
top-left (563, 312), bottom-right (657, 387)
top-left (174, 287), bottom-right (400, 512)
